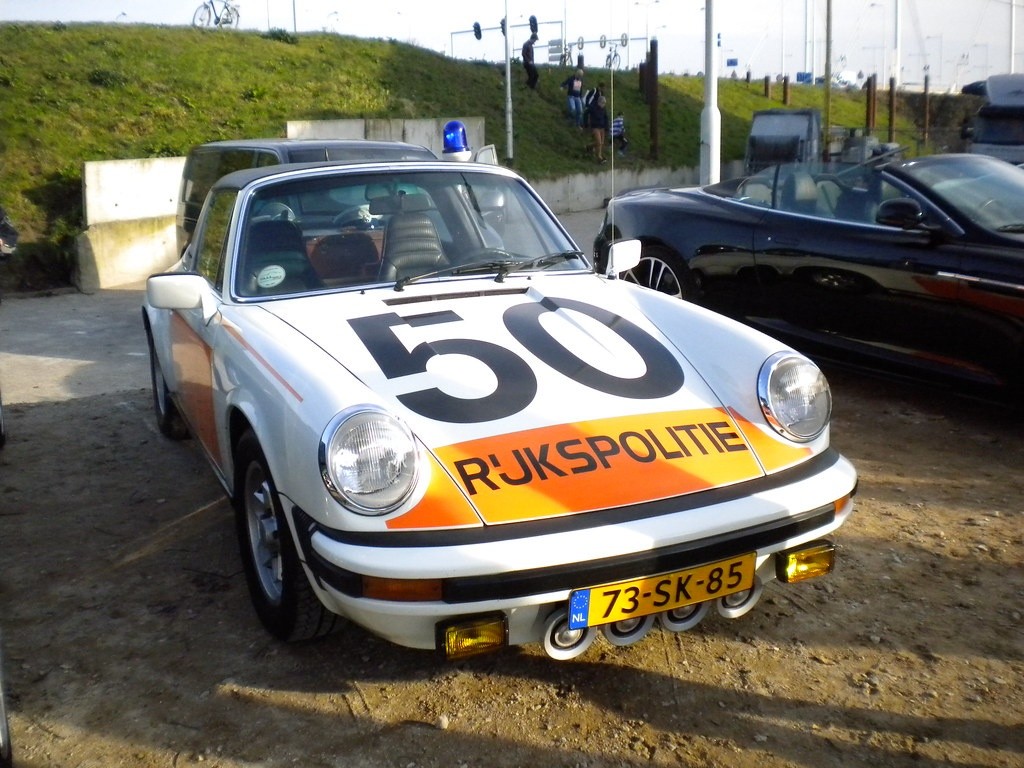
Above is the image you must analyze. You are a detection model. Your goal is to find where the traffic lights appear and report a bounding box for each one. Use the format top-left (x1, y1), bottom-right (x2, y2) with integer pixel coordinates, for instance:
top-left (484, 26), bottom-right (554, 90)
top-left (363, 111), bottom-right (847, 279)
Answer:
top-left (529, 15), bottom-right (538, 33)
top-left (473, 22), bottom-right (481, 39)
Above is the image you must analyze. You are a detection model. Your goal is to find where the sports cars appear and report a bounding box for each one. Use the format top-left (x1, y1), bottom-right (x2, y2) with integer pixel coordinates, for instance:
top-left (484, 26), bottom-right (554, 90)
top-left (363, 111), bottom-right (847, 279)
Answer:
top-left (592, 153), bottom-right (1024, 414)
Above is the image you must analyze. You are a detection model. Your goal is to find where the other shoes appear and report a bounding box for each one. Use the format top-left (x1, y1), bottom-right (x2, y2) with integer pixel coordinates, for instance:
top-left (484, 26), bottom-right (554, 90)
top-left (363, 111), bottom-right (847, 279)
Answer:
top-left (616, 151), bottom-right (624, 158)
top-left (586, 145), bottom-right (595, 158)
top-left (598, 158), bottom-right (607, 166)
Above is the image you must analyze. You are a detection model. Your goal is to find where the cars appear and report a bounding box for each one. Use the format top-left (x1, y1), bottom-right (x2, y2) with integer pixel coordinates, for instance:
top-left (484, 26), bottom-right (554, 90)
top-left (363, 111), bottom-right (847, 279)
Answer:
top-left (141, 120), bottom-right (859, 661)
top-left (962, 80), bottom-right (987, 94)
top-left (802, 77), bottom-right (847, 89)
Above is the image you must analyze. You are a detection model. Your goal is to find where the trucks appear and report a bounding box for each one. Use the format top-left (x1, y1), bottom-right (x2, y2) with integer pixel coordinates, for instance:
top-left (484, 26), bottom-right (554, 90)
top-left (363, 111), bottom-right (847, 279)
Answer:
top-left (960, 73), bottom-right (1024, 164)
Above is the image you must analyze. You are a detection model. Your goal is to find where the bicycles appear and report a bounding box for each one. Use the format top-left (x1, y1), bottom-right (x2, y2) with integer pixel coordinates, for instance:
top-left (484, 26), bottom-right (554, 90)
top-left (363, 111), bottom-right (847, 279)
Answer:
top-left (560, 48), bottom-right (573, 66)
top-left (193, 0), bottom-right (240, 28)
top-left (606, 42), bottom-right (620, 69)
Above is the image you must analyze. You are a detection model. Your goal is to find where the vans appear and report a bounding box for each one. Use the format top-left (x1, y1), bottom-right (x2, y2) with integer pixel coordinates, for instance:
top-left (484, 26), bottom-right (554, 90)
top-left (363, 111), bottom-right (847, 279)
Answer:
top-left (176, 140), bottom-right (509, 262)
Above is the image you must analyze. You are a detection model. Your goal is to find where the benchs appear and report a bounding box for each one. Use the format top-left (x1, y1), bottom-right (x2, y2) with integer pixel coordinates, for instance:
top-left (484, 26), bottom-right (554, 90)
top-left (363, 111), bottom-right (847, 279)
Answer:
top-left (302, 230), bottom-right (387, 283)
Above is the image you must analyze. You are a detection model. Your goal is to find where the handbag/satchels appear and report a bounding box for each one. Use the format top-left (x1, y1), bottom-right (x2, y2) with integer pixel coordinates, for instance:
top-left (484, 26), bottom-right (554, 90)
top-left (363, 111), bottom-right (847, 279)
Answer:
top-left (582, 88), bottom-right (596, 104)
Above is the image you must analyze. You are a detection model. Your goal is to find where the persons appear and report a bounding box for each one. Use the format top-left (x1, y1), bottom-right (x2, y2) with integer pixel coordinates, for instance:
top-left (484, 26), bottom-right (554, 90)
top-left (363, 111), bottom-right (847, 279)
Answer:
top-left (560, 69), bottom-right (585, 130)
top-left (608, 110), bottom-right (629, 156)
top-left (582, 81), bottom-right (608, 165)
top-left (521, 34), bottom-right (539, 88)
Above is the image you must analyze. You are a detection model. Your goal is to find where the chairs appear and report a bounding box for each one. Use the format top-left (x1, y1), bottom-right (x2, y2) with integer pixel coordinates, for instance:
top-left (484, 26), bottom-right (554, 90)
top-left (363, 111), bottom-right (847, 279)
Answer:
top-left (240, 220), bottom-right (324, 298)
top-left (779, 169), bottom-right (818, 216)
top-left (866, 180), bottom-right (901, 223)
top-left (376, 212), bottom-right (451, 283)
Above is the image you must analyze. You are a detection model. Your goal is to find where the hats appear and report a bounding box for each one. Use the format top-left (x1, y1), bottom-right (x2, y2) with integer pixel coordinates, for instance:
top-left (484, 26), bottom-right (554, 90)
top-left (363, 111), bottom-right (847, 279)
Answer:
top-left (255, 200), bottom-right (296, 221)
top-left (333, 204), bottom-right (384, 230)
